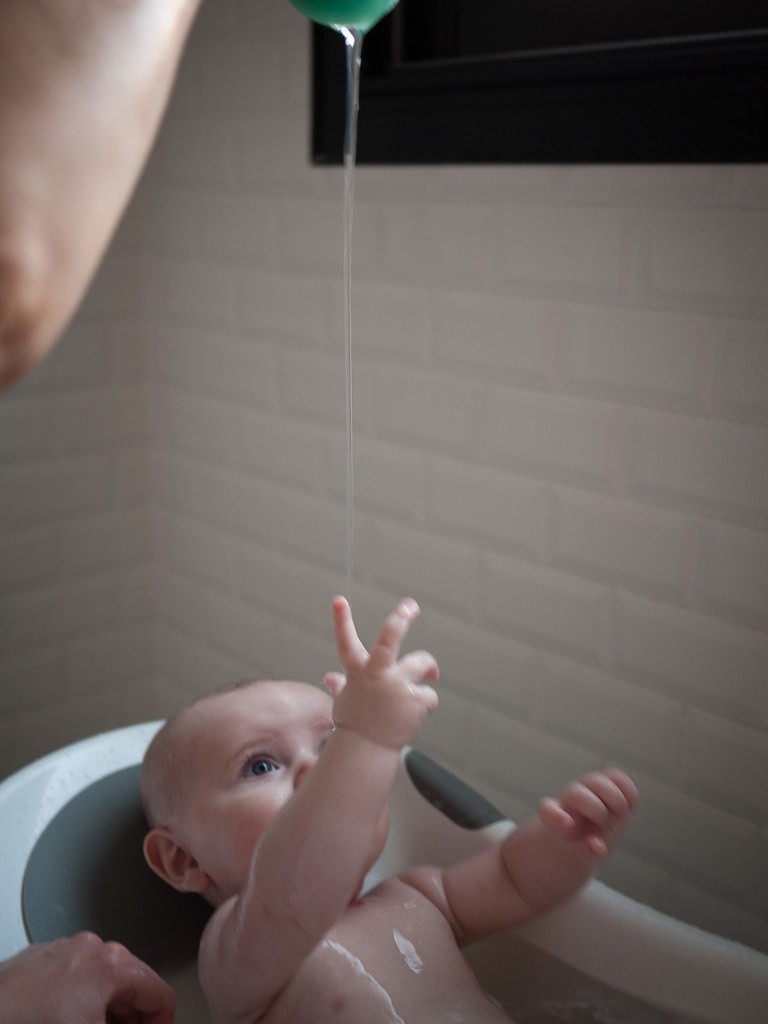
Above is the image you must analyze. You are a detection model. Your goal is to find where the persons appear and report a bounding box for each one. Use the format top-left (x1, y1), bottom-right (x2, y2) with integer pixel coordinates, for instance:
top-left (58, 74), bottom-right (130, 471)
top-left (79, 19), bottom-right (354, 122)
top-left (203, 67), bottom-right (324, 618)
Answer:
top-left (1, 934), bottom-right (188, 1024)
top-left (133, 588), bottom-right (647, 1024)
top-left (1, 0), bottom-right (214, 397)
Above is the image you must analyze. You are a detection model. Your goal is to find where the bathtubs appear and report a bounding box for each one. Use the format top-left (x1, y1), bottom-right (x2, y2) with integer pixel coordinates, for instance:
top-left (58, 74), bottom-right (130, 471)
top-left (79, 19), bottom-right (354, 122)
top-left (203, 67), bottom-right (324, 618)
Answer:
top-left (0, 716), bottom-right (768, 1024)
